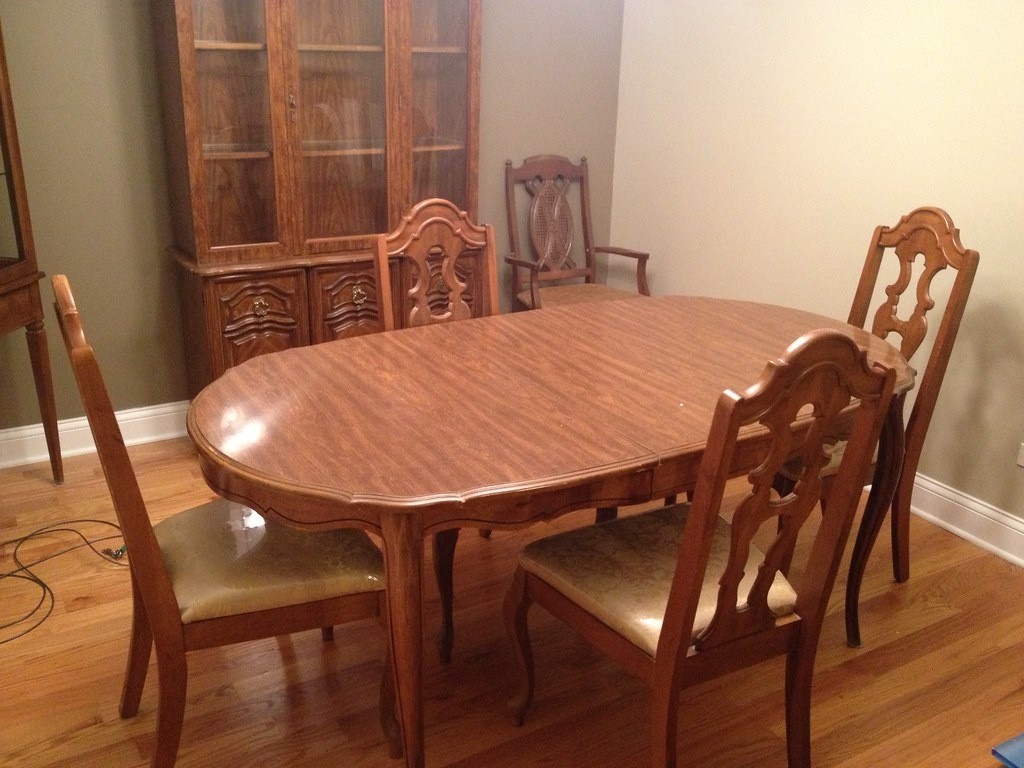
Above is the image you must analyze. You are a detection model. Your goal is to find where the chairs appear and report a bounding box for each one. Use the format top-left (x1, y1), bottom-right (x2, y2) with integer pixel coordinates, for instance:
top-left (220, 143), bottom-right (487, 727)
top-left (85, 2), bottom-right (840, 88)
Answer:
top-left (50, 271), bottom-right (391, 768)
top-left (504, 328), bottom-right (895, 768)
top-left (765, 204), bottom-right (983, 583)
top-left (505, 156), bottom-right (650, 311)
top-left (376, 199), bottom-right (503, 665)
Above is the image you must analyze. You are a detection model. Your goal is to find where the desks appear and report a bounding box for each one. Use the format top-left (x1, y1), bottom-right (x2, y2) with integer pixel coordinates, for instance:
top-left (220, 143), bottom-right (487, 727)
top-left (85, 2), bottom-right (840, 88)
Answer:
top-left (185, 293), bottom-right (917, 767)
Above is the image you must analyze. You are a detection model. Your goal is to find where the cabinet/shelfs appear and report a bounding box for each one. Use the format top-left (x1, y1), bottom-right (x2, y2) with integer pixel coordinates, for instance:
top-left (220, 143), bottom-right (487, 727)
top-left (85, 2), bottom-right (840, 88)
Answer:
top-left (148, 0), bottom-right (481, 402)
